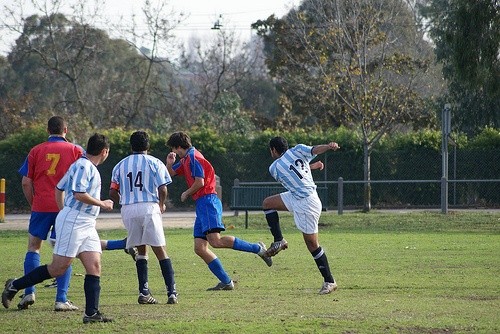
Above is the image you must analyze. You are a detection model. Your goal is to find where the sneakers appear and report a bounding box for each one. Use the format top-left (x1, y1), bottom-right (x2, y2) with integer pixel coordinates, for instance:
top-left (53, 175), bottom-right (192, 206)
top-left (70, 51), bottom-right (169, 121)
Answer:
top-left (44, 279), bottom-right (70, 288)
top-left (1, 278), bottom-right (18, 309)
top-left (319, 280), bottom-right (337, 295)
top-left (124, 237), bottom-right (138, 261)
top-left (55, 300), bottom-right (78, 311)
top-left (207, 280), bottom-right (235, 290)
top-left (83, 311), bottom-right (114, 324)
top-left (137, 293), bottom-right (160, 304)
top-left (266, 238), bottom-right (288, 257)
top-left (167, 293), bottom-right (178, 304)
top-left (17, 293), bottom-right (35, 309)
top-left (257, 241), bottom-right (272, 267)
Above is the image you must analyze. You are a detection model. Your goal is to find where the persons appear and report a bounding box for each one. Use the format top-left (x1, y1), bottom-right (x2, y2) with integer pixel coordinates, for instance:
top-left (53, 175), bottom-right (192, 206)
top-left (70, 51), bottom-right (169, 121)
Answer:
top-left (165, 133), bottom-right (272, 293)
top-left (262, 136), bottom-right (340, 295)
top-left (2, 116), bottom-right (179, 323)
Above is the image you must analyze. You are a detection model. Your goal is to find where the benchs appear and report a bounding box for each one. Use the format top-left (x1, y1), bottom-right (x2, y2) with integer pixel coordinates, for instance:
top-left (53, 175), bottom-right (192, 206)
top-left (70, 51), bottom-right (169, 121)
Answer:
top-left (229, 185), bottom-right (328, 229)
